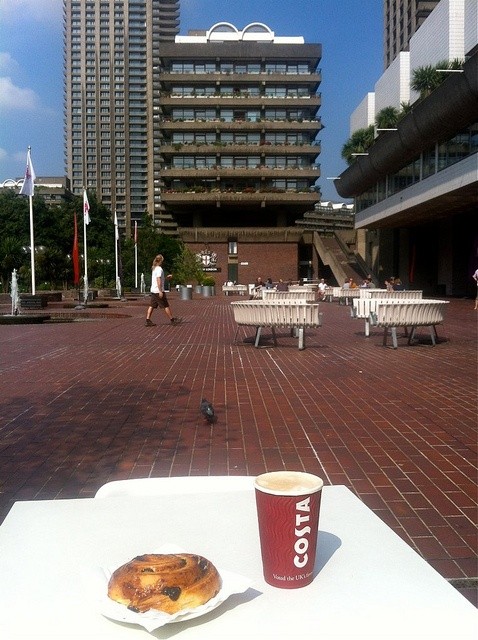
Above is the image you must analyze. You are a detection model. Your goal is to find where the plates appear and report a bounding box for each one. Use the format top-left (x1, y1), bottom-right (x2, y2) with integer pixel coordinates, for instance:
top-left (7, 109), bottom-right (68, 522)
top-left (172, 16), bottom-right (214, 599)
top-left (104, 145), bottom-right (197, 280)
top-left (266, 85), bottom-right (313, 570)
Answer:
top-left (94, 570), bottom-right (237, 623)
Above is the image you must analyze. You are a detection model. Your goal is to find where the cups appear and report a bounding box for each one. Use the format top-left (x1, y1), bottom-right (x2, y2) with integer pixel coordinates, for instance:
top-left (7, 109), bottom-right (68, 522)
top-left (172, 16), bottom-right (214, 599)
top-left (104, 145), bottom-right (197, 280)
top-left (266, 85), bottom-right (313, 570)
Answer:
top-left (255, 471), bottom-right (324, 589)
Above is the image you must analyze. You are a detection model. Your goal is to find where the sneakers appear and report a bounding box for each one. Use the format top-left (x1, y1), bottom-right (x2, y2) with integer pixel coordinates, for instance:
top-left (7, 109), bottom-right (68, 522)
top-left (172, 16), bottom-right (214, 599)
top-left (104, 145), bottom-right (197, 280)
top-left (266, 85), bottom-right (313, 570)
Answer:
top-left (144, 319), bottom-right (156, 326)
top-left (171, 317), bottom-right (182, 325)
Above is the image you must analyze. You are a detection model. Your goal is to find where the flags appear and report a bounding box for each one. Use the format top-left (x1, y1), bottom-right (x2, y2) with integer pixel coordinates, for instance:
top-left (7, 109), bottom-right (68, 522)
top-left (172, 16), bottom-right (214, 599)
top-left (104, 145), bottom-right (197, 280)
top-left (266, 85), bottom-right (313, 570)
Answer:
top-left (81, 184), bottom-right (93, 227)
top-left (112, 208), bottom-right (121, 244)
top-left (16, 144), bottom-right (37, 199)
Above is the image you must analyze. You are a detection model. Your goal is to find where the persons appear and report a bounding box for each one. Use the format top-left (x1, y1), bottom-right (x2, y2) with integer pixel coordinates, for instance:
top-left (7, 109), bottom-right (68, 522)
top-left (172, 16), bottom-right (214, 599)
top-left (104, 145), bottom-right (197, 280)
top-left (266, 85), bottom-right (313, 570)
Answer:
top-left (472, 266), bottom-right (478, 311)
top-left (251, 276), bottom-right (266, 298)
top-left (394, 279), bottom-right (403, 290)
top-left (316, 278), bottom-right (330, 302)
top-left (349, 278), bottom-right (357, 289)
top-left (364, 281), bottom-right (375, 290)
top-left (386, 277), bottom-right (396, 290)
top-left (276, 278), bottom-right (288, 291)
top-left (262, 278), bottom-right (275, 289)
top-left (343, 279), bottom-right (350, 289)
top-left (143, 254), bottom-right (182, 328)
top-left (358, 278), bottom-right (367, 287)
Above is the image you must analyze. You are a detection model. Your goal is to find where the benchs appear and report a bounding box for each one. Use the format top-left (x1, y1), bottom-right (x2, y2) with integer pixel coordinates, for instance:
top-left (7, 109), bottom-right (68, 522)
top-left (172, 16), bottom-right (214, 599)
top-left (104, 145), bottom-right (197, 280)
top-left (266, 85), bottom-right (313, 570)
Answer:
top-left (324, 287), bottom-right (340, 302)
top-left (222, 282), bottom-right (247, 297)
top-left (353, 296), bottom-right (450, 350)
top-left (368, 290), bottom-right (424, 300)
top-left (331, 285), bottom-right (361, 307)
top-left (230, 299), bottom-right (322, 350)
top-left (357, 288), bottom-right (386, 300)
top-left (286, 285), bottom-right (312, 292)
top-left (248, 284), bottom-right (255, 297)
top-left (253, 287), bottom-right (263, 299)
top-left (259, 289), bottom-right (316, 303)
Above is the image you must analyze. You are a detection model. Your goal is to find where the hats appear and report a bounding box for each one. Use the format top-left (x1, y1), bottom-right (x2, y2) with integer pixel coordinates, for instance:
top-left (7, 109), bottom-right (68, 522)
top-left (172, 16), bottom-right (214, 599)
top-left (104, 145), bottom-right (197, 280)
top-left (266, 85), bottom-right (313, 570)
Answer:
top-left (153, 253), bottom-right (164, 266)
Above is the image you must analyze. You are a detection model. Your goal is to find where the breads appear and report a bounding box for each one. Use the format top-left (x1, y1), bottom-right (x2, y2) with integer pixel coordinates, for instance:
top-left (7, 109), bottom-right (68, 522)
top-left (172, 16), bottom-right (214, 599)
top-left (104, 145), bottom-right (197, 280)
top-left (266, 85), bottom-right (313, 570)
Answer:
top-left (107, 552), bottom-right (222, 613)
